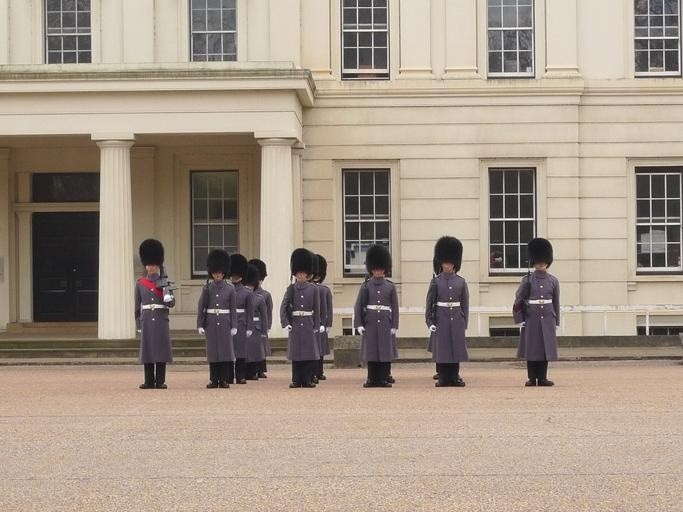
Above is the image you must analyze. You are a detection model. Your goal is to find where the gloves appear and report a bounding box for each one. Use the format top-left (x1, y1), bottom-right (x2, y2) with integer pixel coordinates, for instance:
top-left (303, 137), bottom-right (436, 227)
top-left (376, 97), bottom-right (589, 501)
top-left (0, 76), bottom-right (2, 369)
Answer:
top-left (357, 326), bottom-right (366, 336)
top-left (518, 321), bottom-right (526, 328)
top-left (390, 327), bottom-right (396, 336)
top-left (230, 327), bottom-right (238, 336)
top-left (319, 325), bottom-right (325, 333)
top-left (428, 324), bottom-right (437, 332)
top-left (163, 294), bottom-right (174, 303)
top-left (285, 324), bottom-right (293, 332)
top-left (198, 327), bottom-right (206, 336)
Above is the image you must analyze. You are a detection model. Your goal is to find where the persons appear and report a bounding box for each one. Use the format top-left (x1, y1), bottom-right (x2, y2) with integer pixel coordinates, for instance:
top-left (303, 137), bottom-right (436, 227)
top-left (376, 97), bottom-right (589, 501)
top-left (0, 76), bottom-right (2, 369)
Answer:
top-left (197, 250), bottom-right (273, 389)
top-left (353, 244), bottom-right (399, 388)
top-left (279, 247), bottom-right (333, 390)
top-left (512, 238), bottom-right (560, 386)
top-left (134, 239), bottom-right (175, 386)
top-left (424, 236), bottom-right (469, 388)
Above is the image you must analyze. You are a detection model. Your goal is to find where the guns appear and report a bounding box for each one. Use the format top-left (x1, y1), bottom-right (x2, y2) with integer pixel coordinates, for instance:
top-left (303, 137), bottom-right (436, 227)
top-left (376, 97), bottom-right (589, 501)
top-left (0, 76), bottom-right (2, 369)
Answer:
top-left (286, 276), bottom-right (295, 324)
top-left (360, 274), bottom-right (369, 335)
top-left (523, 271), bottom-right (531, 321)
top-left (429, 274), bottom-right (437, 324)
top-left (157, 265), bottom-right (179, 302)
top-left (201, 276), bottom-right (209, 327)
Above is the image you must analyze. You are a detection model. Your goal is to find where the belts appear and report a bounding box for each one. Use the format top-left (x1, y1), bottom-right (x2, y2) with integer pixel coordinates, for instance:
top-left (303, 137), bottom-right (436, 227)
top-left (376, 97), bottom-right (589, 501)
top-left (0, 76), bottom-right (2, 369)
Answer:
top-left (527, 298), bottom-right (553, 305)
top-left (365, 303), bottom-right (391, 312)
top-left (141, 304), bottom-right (169, 310)
top-left (290, 310), bottom-right (314, 317)
top-left (236, 307), bottom-right (245, 314)
top-left (252, 316), bottom-right (260, 322)
top-left (206, 308), bottom-right (230, 315)
top-left (436, 301), bottom-right (461, 308)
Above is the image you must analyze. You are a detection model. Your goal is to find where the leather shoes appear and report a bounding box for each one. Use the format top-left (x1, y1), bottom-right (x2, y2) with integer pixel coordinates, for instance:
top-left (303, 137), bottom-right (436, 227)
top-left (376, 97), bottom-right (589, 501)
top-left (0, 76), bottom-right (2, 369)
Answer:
top-left (155, 382), bottom-right (166, 390)
top-left (525, 378), bottom-right (538, 387)
top-left (139, 381), bottom-right (156, 390)
top-left (537, 377), bottom-right (555, 387)
top-left (206, 371), bottom-right (469, 396)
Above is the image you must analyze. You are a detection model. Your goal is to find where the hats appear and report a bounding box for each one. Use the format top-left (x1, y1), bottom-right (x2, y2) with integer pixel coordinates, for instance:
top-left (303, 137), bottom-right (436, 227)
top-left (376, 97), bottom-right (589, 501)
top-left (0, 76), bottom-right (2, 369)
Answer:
top-left (139, 238), bottom-right (165, 266)
top-left (526, 238), bottom-right (556, 267)
top-left (207, 236), bottom-right (466, 294)
